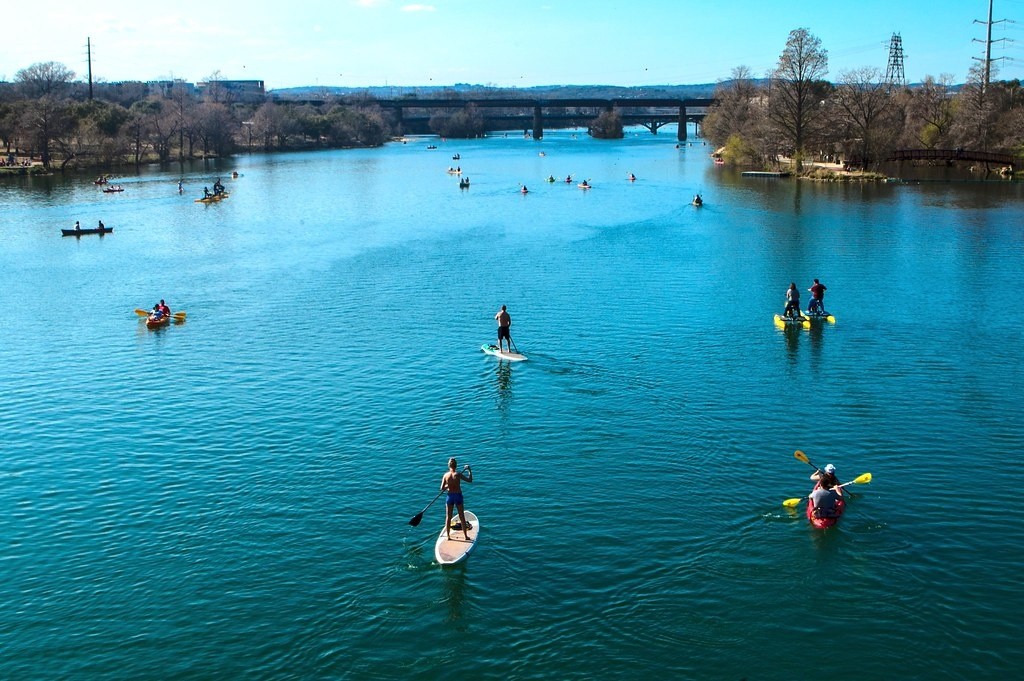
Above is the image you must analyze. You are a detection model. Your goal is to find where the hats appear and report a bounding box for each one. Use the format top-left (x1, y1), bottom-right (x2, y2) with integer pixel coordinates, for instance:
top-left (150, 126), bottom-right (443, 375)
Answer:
top-left (824, 464), bottom-right (836, 473)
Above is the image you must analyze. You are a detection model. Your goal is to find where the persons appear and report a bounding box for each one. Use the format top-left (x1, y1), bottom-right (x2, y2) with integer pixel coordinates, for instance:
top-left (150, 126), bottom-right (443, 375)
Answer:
top-left (809, 476), bottom-right (843, 519)
top-left (428, 128), bottom-right (725, 202)
top-left (98, 176), bottom-right (120, 190)
top-left (495, 305), bottom-right (511, 353)
top-left (782, 282), bottom-right (801, 318)
top-left (159, 299), bottom-right (170, 317)
top-left (150, 304), bottom-right (164, 320)
top-left (805, 279), bottom-right (827, 313)
top-left (98, 221), bottom-right (104, 228)
top-left (811, 464), bottom-right (840, 489)
top-left (75, 221), bottom-right (80, 229)
top-left (0, 154), bottom-right (31, 166)
top-left (441, 458), bottom-right (472, 540)
top-left (178, 177), bottom-right (225, 199)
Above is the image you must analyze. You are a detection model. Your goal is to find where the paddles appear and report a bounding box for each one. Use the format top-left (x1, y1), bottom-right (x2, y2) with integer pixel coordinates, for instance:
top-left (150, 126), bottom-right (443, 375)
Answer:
top-left (793, 449), bottom-right (855, 497)
top-left (783, 472), bottom-right (872, 508)
top-left (587, 179), bottom-right (591, 182)
top-left (509, 336), bottom-right (517, 352)
top-left (408, 466), bottom-right (469, 528)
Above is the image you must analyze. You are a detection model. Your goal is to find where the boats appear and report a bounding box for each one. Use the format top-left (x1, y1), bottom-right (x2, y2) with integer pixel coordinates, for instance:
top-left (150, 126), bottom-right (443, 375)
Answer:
top-left (102, 189), bottom-right (125, 192)
top-left (578, 184), bottom-right (591, 188)
top-left (714, 160), bottom-right (725, 164)
top-left (61, 227), bottom-right (113, 236)
top-left (448, 171), bottom-right (462, 173)
top-left (693, 201), bottom-right (702, 206)
top-left (195, 192), bottom-right (231, 202)
top-left (460, 183), bottom-right (470, 188)
top-left (807, 479), bottom-right (844, 530)
top-left (146, 313), bottom-right (169, 329)
top-left (521, 190), bottom-right (528, 193)
top-left (774, 308), bottom-right (836, 331)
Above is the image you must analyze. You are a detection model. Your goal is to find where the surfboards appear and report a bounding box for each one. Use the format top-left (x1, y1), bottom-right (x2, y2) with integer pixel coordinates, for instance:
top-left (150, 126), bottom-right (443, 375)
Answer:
top-left (481, 344), bottom-right (528, 361)
top-left (435, 510), bottom-right (480, 565)
